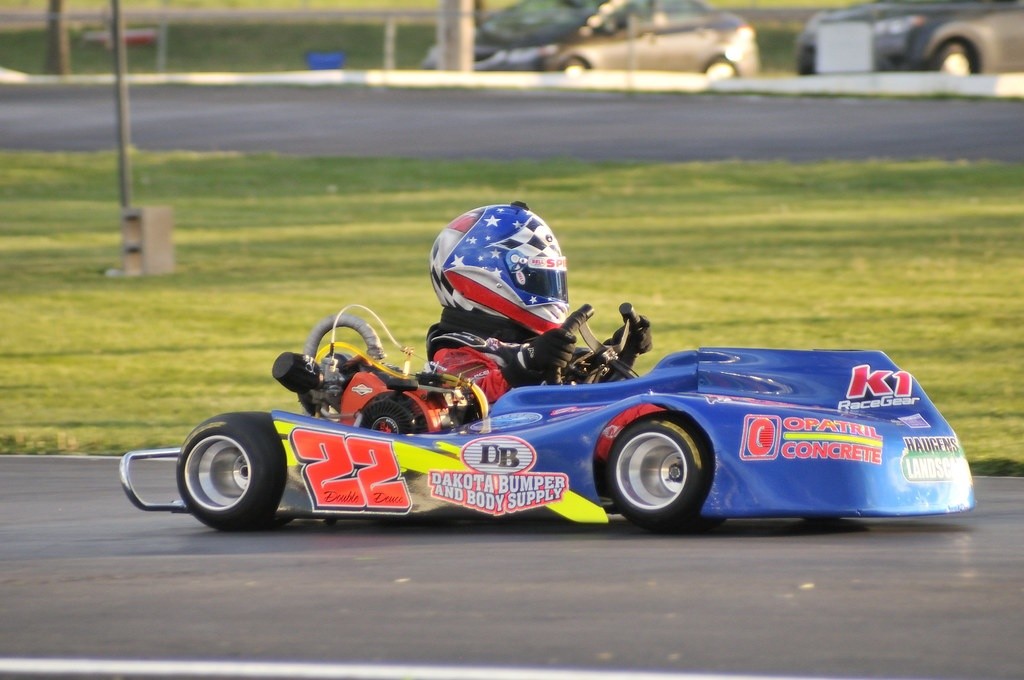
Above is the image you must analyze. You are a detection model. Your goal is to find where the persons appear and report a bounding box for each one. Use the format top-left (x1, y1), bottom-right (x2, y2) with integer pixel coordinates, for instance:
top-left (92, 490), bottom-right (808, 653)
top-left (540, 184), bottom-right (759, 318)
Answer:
top-left (424, 201), bottom-right (652, 418)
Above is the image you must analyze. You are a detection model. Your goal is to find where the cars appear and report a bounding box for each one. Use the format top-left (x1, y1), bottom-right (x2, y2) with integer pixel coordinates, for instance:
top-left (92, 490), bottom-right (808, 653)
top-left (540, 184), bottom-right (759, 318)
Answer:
top-left (422, 0), bottom-right (757, 77)
top-left (794, 0), bottom-right (1024, 76)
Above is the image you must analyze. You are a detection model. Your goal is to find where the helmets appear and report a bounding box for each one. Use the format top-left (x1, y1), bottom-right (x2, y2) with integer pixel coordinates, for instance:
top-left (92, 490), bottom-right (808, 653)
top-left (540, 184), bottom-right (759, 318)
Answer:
top-left (428, 201), bottom-right (568, 334)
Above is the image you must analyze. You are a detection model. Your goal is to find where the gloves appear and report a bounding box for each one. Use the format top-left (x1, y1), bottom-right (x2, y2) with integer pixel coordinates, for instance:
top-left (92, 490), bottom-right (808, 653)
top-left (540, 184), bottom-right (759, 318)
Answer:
top-left (613, 316), bottom-right (652, 355)
top-left (522, 328), bottom-right (577, 371)
